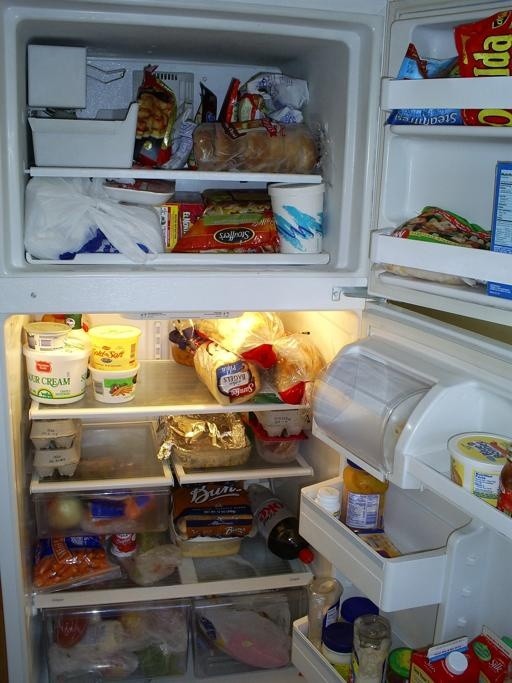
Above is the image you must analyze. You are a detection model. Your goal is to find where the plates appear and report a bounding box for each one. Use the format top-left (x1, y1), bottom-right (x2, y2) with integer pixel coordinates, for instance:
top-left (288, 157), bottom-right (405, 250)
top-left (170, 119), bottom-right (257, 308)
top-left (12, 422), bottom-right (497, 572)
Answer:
top-left (101, 185), bottom-right (175, 207)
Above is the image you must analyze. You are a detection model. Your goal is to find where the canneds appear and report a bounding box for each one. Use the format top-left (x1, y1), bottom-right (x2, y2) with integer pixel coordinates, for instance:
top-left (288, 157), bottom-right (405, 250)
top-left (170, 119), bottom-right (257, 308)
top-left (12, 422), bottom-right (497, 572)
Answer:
top-left (339, 458), bottom-right (389, 531)
top-left (349, 614), bottom-right (392, 683)
top-left (382, 647), bottom-right (413, 683)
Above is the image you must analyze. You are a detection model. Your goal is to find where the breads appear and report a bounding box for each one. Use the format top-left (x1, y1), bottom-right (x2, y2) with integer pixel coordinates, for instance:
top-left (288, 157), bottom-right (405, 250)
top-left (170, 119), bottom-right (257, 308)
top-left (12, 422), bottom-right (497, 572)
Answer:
top-left (195, 128), bottom-right (315, 172)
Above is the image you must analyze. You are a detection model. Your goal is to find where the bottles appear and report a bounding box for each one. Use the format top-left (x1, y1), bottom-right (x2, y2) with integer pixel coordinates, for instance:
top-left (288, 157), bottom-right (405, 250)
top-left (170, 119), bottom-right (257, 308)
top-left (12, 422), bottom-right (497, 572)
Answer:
top-left (248, 482), bottom-right (316, 565)
top-left (306, 577), bottom-right (478, 683)
top-left (496, 451), bottom-right (512, 518)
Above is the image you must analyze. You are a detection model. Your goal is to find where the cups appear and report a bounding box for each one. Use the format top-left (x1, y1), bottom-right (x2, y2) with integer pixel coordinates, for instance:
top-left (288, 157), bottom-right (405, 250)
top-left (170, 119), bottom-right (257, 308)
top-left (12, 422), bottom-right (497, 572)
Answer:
top-left (268, 183), bottom-right (325, 256)
top-left (23, 324), bottom-right (141, 405)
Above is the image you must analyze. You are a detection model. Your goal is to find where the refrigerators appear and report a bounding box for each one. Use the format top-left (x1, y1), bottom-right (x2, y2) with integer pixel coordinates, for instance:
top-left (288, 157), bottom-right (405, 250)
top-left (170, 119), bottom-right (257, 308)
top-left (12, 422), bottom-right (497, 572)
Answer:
top-left (1, 1), bottom-right (511, 683)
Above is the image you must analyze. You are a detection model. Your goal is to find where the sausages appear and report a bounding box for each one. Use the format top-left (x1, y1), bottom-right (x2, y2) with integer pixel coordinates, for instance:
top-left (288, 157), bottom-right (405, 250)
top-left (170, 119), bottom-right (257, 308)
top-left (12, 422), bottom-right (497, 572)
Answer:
top-left (35, 549), bottom-right (110, 589)
